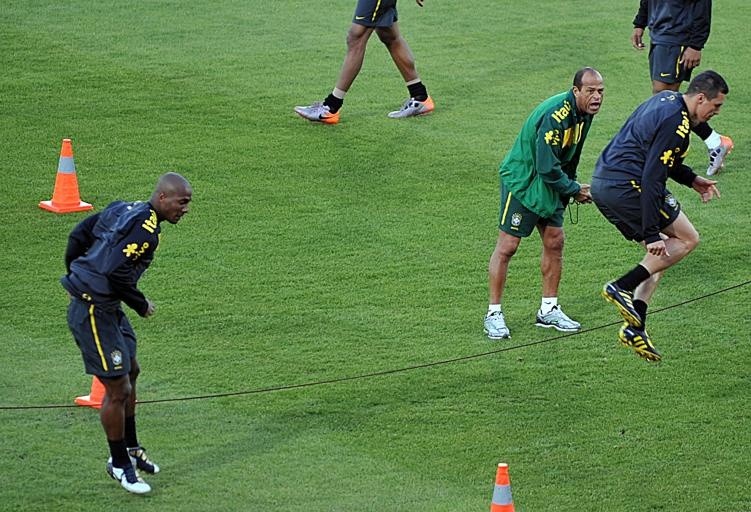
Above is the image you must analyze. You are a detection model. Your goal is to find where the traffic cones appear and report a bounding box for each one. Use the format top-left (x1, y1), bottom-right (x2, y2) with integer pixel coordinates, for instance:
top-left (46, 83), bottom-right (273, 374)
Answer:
top-left (37, 137), bottom-right (95, 214)
top-left (74, 375), bottom-right (106, 409)
top-left (489, 462), bottom-right (520, 512)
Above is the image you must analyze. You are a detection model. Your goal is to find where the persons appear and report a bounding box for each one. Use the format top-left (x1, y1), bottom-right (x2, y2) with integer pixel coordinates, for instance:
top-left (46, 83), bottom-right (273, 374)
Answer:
top-left (632, 0), bottom-right (734, 174)
top-left (481, 67), bottom-right (605, 341)
top-left (58, 172), bottom-right (191, 495)
top-left (591, 70), bottom-right (729, 362)
top-left (292, 1), bottom-right (434, 125)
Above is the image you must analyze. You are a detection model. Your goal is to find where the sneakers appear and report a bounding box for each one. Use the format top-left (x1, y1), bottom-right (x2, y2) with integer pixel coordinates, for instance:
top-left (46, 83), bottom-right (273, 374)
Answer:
top-left (484, 311), bottom-right (511, 340)
top-left (106, 454), bottom-right (152, 493)
top-left (126, 446), bottom-right (160, 475)
top-left (295, 102), bottom-right (341, 125)
top-left (707, 136), bottom-right (735, 176)
top-left (388, 95), bottom-right (435, 120)
top-left (536, 305), bottom-right (583, 332)
top-left (619, 321), bottom-right (663, 361)
top-left (602, 278), bottom-right (642, 327)
